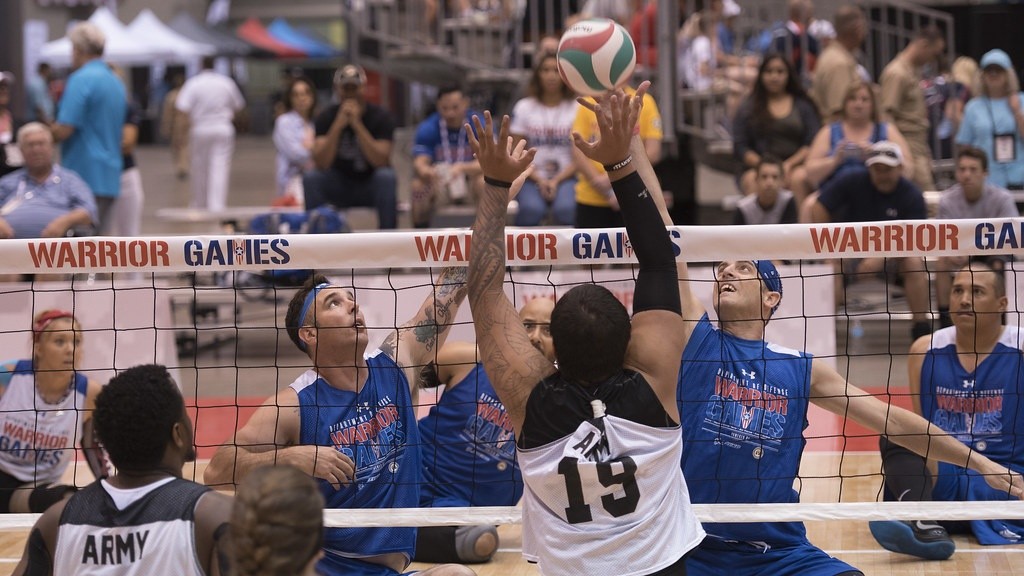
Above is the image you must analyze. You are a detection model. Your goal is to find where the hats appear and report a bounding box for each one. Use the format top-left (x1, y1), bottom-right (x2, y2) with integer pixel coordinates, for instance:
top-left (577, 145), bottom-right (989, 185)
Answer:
top-left (864, 139), bottom-right (906, 168)
top-left (333, 63), bottom-right (367, 87)
top-left (67, 21), bottom-right (105, 56)
top-left (949, 56), bottom-right (979, 97)
top-left (979, 49), bottom-right (1010, 74)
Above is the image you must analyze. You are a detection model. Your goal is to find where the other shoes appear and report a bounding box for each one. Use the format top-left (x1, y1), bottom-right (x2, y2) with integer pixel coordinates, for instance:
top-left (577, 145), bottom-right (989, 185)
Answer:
top-left (910, 323), bottom-right (933, 341)
top-left (934, 306), bottom-right (953, 327)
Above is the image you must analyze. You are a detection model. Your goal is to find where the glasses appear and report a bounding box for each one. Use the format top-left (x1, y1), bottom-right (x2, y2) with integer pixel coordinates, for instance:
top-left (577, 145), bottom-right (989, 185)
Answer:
top-left (866, 149), bottom-right (901, 160)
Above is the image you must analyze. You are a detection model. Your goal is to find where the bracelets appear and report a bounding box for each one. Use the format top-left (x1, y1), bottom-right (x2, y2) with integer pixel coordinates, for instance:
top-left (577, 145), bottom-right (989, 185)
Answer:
top-left (603, 154), bottom-right (632, 172)
top-left (484, 175), bottom-right (511, 188)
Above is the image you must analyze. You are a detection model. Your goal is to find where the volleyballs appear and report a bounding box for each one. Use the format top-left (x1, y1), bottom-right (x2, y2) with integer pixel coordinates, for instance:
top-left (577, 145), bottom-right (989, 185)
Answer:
top-left (556, 17), bottom-right (636, 97)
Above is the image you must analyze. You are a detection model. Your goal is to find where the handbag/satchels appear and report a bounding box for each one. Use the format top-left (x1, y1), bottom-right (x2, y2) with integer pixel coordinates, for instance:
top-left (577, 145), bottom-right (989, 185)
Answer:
top-left (246, 204), bottom-right (354, 235)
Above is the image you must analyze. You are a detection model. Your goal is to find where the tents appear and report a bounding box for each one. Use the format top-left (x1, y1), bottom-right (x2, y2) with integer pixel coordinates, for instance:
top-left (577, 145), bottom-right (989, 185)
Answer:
top-left (40, 7), bottom-right (343, 139)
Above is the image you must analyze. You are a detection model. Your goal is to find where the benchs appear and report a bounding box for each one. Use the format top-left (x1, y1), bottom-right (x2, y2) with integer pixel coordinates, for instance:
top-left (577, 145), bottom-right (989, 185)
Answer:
top-left (158, 201), bottom-right (521, 223)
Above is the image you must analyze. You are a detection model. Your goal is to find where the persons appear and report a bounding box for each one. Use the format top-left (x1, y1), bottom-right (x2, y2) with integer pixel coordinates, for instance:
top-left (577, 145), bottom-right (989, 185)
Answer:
top-left (303, 64), bottom-right (398, 230)
top-left (407, 0), bottom-right (1024, 228)
top-left (0, 26), bottom-right (318, 237)
top-left (0, 78), bottom-right (1024, 576)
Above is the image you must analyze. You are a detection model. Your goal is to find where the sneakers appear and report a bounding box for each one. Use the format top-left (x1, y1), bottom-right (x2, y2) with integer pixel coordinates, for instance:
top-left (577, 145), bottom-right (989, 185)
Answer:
top-left (869, 520), bottom-right (955, 560)
